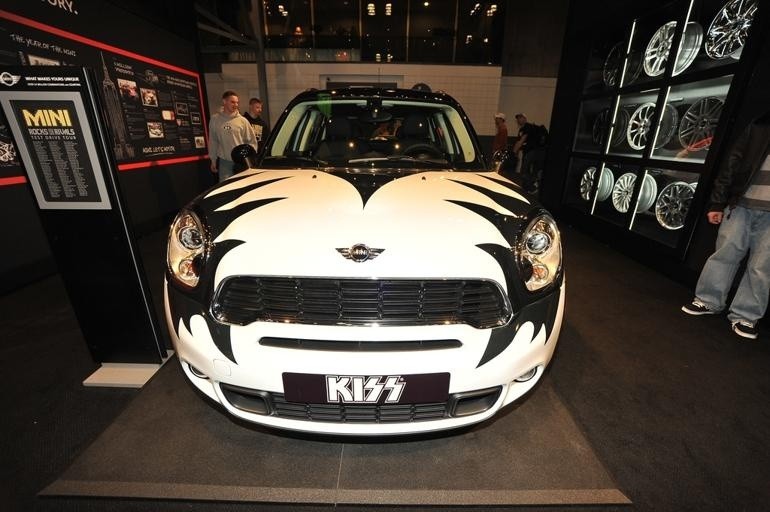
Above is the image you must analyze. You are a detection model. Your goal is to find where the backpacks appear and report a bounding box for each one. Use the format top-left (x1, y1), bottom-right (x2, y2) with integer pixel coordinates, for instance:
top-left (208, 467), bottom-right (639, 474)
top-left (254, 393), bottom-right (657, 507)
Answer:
top-left (530, 124), bottom-right (548, 158)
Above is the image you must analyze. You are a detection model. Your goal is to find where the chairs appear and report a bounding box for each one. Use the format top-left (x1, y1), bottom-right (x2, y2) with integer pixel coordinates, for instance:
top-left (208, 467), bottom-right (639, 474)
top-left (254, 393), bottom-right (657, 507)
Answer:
top-left (314, 116), bottom-right (441, 160)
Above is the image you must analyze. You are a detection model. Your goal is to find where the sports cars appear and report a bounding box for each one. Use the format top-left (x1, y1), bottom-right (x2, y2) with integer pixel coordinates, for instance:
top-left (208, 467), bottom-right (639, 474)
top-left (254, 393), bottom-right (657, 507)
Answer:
top-left (164, 80), bottom-right (570, 443)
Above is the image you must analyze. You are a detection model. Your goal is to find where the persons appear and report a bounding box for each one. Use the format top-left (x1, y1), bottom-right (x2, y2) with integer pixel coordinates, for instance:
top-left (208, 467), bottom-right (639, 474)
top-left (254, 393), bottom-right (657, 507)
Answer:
top-left (676, 110), bottom-right (769, 345)
top-left (514, 113), bottom-right (546, 196)
top-left (490, 115), bottom-right (510, 175)
top-left (209, 89), bottom-right (261, 185)
top-left (240, 97), bottom-right (272, 157)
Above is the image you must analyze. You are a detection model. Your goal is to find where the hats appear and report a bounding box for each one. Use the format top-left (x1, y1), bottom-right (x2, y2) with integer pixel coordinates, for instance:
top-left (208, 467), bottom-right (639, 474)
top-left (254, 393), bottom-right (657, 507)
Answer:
top-left (493, 112), bottom-right (506, 119)
top-left (514, 112), bottom-right (527, 118)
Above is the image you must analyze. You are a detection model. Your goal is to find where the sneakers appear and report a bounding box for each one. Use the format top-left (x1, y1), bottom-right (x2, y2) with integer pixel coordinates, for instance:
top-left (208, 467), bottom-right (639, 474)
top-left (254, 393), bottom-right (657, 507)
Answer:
top-left (682, 301), bottom-right (717, 315)
top-left (732, 319), bottom-right (759, 339)
